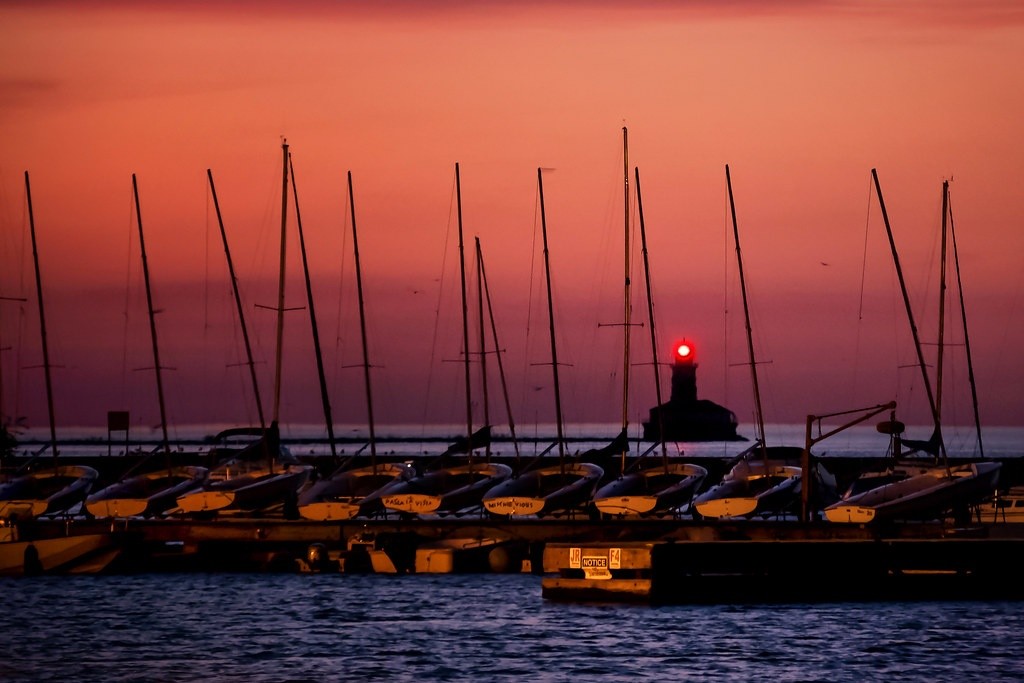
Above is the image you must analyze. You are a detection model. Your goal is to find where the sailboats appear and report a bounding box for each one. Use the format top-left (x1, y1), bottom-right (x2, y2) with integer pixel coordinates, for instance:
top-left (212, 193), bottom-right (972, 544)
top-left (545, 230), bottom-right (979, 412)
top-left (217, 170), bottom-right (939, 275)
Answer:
top-left (2, 127), bottom-right (1023, 592)
